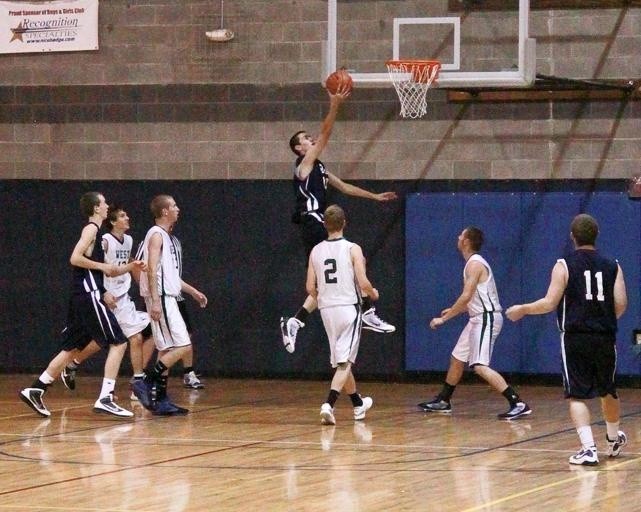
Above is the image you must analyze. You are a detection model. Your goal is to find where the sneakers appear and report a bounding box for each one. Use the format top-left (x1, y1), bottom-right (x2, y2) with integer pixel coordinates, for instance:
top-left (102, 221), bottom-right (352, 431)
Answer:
top-left (498, 401), bottom-right (532, 419)
top-left (320, 402), bottom-right (336, 425)
top-left (361, 307), bottom-right (396, 334)
top-left (417, 397), bottom-right (452, 413)
top-left (19, 366), bottom-right (205, 417)
top-left (280, 316), bottom-right (305, 354)
top-left (607, 431), bottom-right (627, 457)
top-left (353, 396), bottom-right (373, 420)
top-left (569, 446), bottom-right (599, 465)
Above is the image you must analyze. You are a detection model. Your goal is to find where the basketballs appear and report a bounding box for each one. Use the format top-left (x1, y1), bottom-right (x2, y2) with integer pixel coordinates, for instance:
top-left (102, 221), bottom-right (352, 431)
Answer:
top-left (327, 71), bottom-right (354, 95)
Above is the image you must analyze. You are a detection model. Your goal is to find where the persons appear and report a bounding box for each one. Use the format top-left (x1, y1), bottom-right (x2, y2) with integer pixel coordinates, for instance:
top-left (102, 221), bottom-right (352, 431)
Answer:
top-left (306, 204), bottom-right (379, 426)
top-left (18, 191), bottom-right (134, 417)
top-left (135, 218), bottom-right (206, 389)
top-left (61, 202), bottom-right (157, 400)
top-left (417, 224), bottom-right (533, 420)
top-left (505, 214), bottom-right (628, 467)
top-left (280, 83), bottom-right (394, 352)
top-left (130, 194), bottom-right (208, 416)
